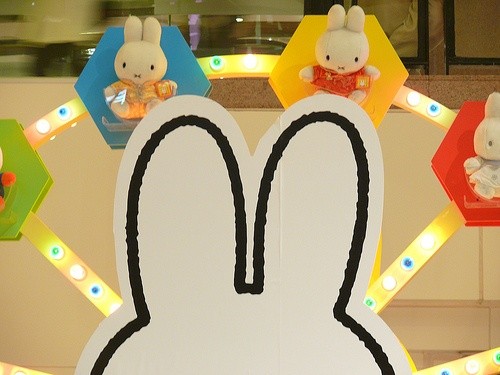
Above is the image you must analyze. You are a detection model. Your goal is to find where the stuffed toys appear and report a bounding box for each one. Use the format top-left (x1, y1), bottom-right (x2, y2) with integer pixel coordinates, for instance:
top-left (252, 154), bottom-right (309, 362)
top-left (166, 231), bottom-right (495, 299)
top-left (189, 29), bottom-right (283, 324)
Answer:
top-left (298, 3), bottom-right (380, 103)
top-left (463, 91), bottom-right (500, 199)
top-left (104, 14), bottom-right (178, 121)
